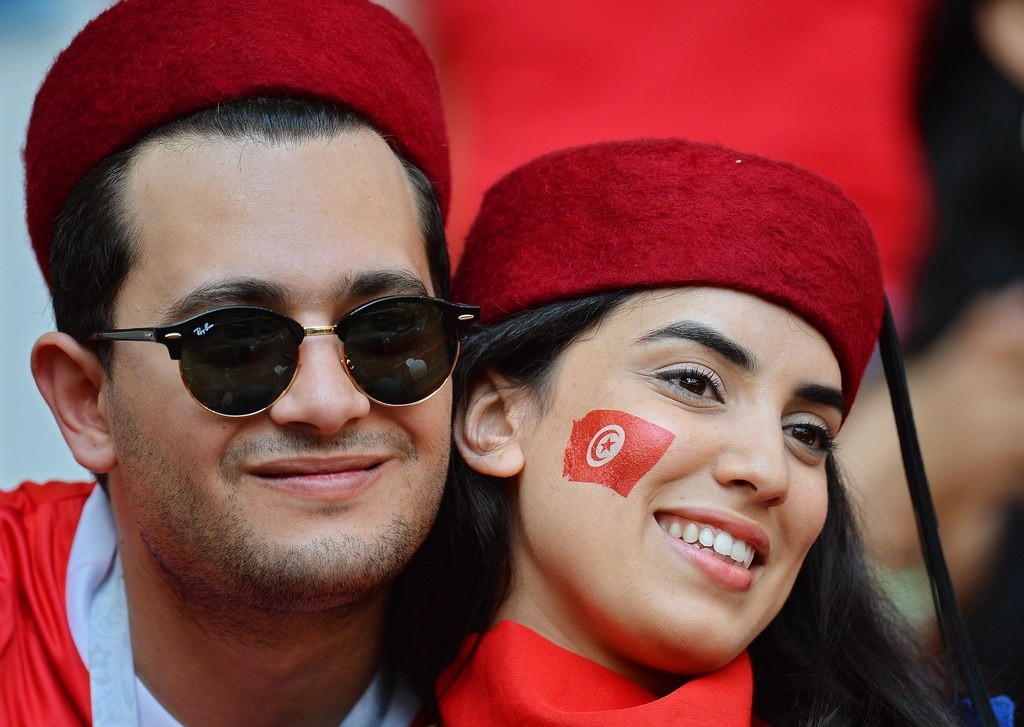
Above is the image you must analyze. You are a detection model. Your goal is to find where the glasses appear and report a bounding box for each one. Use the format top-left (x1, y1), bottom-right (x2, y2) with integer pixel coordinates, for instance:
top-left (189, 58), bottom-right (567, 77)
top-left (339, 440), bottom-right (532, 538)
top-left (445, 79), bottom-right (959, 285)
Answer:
top-left (91, 293), bottom-right (482, 418)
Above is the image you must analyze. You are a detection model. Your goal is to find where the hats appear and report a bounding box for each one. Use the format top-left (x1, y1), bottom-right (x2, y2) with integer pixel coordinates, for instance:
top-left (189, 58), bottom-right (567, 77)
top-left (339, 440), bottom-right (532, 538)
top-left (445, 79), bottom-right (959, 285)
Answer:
top-left (453, 136), bottom-right (884, 431)
top-left (22, 0), bottom-right (450, 275)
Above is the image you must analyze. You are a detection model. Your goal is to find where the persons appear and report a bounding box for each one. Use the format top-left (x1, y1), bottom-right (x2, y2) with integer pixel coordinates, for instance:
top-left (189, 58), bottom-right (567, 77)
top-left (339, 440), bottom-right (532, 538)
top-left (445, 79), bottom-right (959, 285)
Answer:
top-left (388, 138), bottom-right (974, 727)
top-left (0, 0), bottom-right (482, 727)
